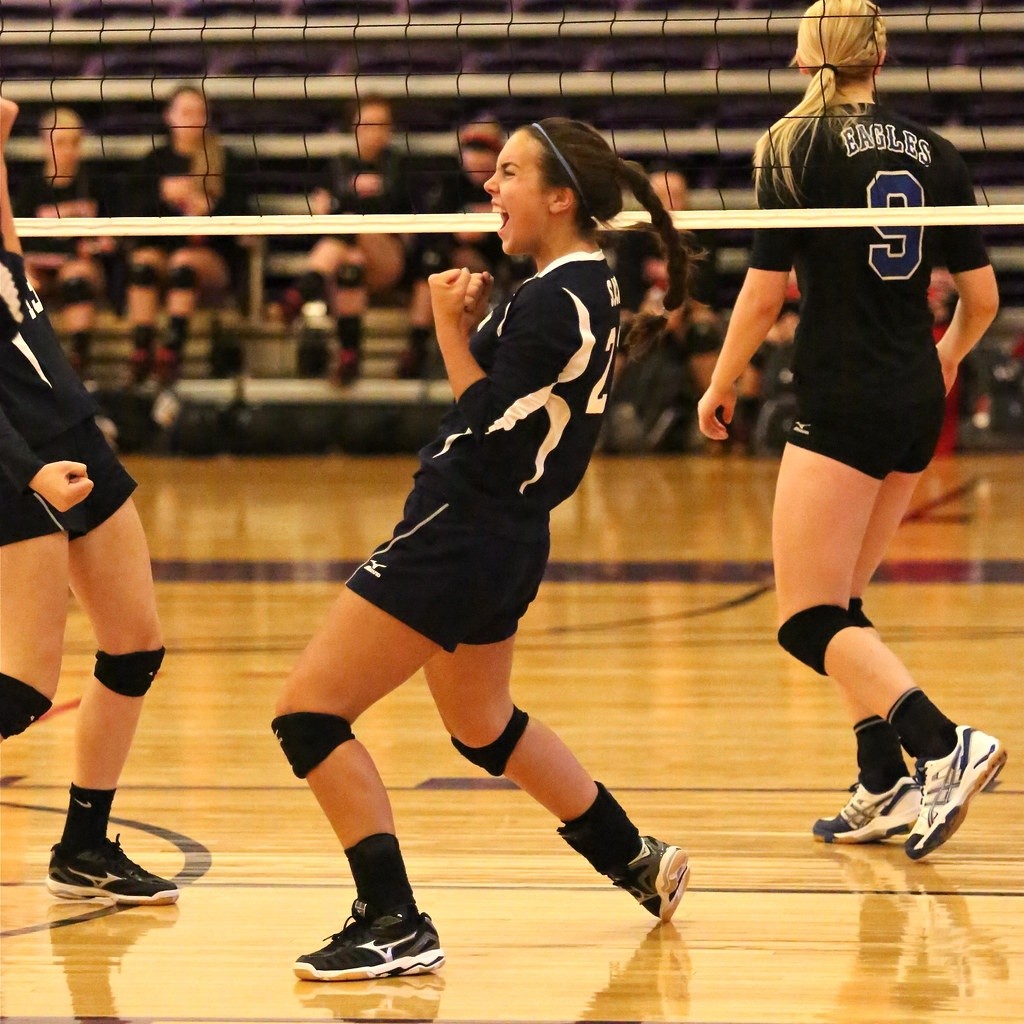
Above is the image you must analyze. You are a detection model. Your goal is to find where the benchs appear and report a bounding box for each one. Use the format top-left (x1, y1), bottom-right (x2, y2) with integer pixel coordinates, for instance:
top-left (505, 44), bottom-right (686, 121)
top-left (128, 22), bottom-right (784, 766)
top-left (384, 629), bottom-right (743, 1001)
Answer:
top-left (36, 302), bottom-right (1023, 381)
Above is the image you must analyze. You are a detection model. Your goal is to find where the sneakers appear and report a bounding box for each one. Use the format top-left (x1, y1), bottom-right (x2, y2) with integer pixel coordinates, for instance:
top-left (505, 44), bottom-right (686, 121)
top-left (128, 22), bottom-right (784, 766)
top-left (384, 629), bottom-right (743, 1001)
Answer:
top-left (622, 919), bottom-right (691, 1024)
top-left (46, 906), bottom-right (178, 964)
top-left (294, 975), bottom-right (446, 1022)
top-left (608, 839), bottom-right (691, 922)
top-left (904, 723), bottom-right (1006, 861)
top-left (45, 833), bottom-right (179, 906)
top-left (293, 899), bottom-right (445, 982)
top-left (813, 777), bottom-right (924, 843)
top-left (882, 867), bottom-right (1006, 998)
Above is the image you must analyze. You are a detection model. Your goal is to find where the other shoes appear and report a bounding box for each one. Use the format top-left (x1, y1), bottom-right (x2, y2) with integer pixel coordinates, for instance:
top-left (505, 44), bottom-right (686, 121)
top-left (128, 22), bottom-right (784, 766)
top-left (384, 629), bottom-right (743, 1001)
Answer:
top-left (144, 346), bottom-right (177, 392)
top-left (128, 349), bottom-right (148, 386)
top-left (334, 347), bottom-right (360, 390)
top-left (399, 353), bottom-right (426, 379)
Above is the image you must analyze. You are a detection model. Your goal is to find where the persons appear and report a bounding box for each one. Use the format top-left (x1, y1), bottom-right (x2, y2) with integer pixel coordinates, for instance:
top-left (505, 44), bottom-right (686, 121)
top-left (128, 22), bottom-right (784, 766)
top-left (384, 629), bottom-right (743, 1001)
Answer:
top-left (0, 97), bottom-right (180, 905)
top-left (21, 84), bottom-right (1000, 428)
top-left (697, 0), bottom-right (1008, 860)
top-left (270, 117), bottom-right (689, 981)
